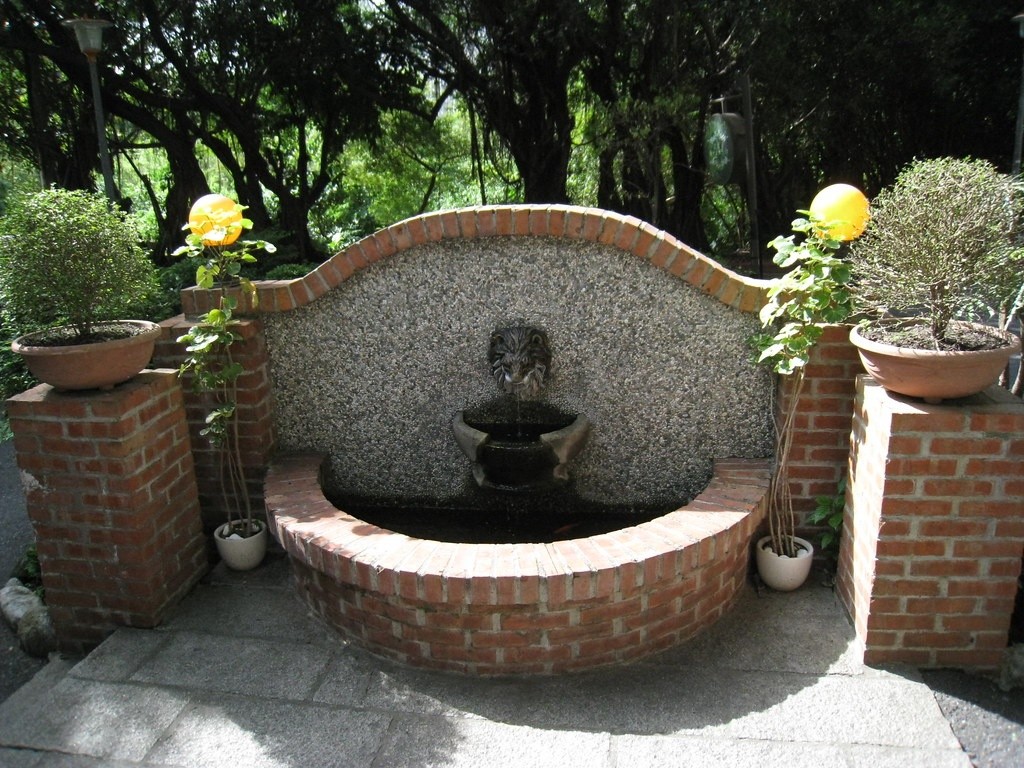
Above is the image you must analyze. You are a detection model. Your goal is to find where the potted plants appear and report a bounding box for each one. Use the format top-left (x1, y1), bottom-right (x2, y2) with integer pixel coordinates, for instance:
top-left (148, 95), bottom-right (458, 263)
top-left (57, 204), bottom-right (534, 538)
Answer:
top-left (3, 185), bottom-right (167, 392)
top-left (848, 148), bottom-right (1024, 403)
top-left (754, 208), bottom-right (852, 592)
top-left (172, 197), bottom-right (276, 571)
